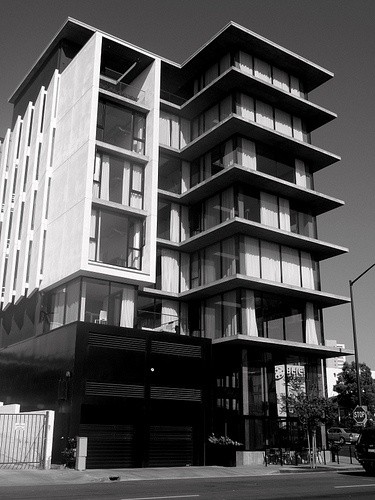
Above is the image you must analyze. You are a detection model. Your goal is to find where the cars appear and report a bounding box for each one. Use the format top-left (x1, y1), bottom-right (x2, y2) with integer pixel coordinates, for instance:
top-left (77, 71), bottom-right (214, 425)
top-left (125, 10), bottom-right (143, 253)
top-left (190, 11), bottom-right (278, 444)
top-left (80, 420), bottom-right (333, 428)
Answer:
top-left (327, 427), bottom-right (360, 445)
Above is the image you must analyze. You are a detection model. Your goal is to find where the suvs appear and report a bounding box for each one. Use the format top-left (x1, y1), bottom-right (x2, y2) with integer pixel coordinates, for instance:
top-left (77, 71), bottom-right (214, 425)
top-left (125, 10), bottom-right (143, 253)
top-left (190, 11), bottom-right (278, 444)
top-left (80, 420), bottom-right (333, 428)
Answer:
top-left (355, 425), bottom-right (375, 477)
